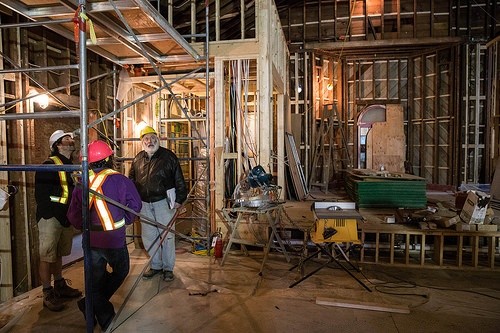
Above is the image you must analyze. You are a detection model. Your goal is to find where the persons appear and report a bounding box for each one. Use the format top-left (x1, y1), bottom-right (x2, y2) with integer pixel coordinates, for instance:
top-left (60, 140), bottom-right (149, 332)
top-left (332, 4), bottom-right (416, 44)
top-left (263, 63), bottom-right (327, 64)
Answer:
top-left (129, 126), bottom-right (187, 281)
top-left (35, 129), bottom-right (82, 312)
top-left (66, 140), bottom-right (143, 330)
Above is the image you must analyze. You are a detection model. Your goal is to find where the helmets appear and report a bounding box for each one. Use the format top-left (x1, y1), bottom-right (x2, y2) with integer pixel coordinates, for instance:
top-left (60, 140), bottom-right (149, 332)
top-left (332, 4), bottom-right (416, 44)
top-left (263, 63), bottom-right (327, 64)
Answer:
top-left (140, 125), bottom-right (157, 139)
top-left (49, 129), bottom-right (74, 151)
top-left (79, 140), bottom-right (113, 163)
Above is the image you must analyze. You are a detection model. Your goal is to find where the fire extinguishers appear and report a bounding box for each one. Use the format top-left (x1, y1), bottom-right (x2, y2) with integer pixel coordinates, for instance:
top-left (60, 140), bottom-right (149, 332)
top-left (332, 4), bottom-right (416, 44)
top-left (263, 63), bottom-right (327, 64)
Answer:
top-left (213, 227), bottom-right (223, 258)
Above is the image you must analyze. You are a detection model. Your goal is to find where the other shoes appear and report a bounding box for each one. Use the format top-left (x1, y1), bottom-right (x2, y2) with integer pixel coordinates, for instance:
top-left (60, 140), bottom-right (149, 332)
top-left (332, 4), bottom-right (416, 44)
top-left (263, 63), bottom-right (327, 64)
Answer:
top-left (143, 268), bottom-right (163, 277)
top-left (78, 297), bottom-right (97, 327)
top-left (163, 271), bottom-right (175, 281)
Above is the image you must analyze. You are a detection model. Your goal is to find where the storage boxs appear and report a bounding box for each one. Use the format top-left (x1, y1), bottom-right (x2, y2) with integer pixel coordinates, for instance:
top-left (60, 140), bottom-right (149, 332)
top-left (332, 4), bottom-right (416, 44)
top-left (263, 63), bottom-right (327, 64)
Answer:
top-left (461, 190), bottom-right (491, 225)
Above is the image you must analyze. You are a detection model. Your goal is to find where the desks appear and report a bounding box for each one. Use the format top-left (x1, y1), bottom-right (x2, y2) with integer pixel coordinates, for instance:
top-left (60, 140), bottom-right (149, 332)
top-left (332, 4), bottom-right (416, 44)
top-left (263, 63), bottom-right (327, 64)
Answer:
top-left (220, 204), bottom-right (292, 276)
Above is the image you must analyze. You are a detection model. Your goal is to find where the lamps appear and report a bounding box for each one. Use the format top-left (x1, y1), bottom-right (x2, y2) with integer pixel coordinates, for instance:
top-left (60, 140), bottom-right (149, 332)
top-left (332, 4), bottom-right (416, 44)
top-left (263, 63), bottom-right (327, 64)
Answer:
top-left (29, 87), bottom-right (49, 110)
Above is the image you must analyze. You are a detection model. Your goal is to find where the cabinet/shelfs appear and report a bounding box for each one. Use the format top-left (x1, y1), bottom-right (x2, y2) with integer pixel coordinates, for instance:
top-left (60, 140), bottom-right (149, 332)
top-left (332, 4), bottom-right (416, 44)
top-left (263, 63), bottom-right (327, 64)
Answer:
top-left (168, 92), bottom-right (209, 241)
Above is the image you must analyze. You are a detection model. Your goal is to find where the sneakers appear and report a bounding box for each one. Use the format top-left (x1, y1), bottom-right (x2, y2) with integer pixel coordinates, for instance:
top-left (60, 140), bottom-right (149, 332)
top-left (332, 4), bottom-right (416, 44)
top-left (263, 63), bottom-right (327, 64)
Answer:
top-left (42, 287), bottom-right (66, 311)
top-left (54, 278), bottom-right (82, 298)
